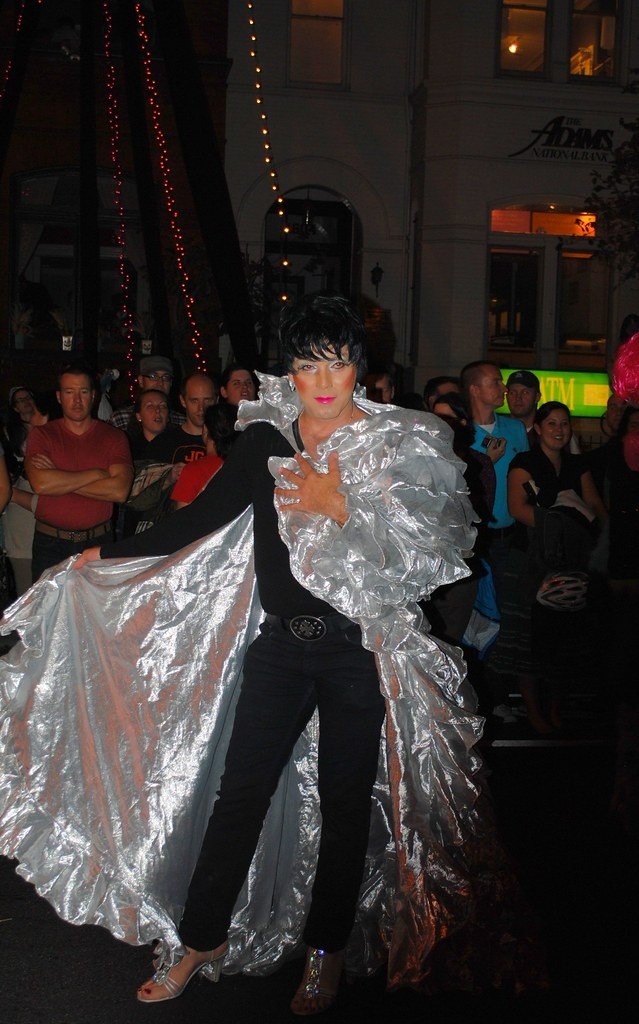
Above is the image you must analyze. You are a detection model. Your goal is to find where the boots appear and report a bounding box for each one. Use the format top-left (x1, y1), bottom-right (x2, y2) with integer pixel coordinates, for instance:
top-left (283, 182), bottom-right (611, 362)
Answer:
top-left (607, 703), bottom-right (639, 833)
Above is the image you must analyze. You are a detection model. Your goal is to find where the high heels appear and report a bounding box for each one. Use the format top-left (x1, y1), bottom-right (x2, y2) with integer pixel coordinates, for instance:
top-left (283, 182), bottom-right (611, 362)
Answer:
top-left (137, 941), bottom-right (231, 1003)
top-left (290, 947), bottom-right (345, 1014)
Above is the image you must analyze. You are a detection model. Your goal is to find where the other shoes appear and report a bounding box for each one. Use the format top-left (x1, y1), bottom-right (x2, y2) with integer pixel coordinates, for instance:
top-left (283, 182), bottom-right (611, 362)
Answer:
top-left (550, 721), bottom-right (580, 742)
top-left (523, 716), bottom-right (560, 738)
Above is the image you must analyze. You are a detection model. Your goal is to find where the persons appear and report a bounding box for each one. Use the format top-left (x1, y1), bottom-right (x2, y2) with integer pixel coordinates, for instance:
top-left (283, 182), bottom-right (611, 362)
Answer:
top-left (0, 314), bottom-right (639, 736)
top-left (47, 290), bottom-right (486, 1018)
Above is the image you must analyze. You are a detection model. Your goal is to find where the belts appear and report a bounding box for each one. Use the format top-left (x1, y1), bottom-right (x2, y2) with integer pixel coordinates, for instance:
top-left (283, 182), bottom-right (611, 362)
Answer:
top-left (266, 615), bottom-right (354, 642)
top-left (35, 519), bottom-right (111, 543)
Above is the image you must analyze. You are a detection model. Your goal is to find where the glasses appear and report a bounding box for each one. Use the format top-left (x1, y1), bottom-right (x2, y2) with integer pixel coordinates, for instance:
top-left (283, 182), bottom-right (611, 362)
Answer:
top-left (14, 395), bottom-right (32, 404)
top-left (142, 373), bottom-right (173, 384)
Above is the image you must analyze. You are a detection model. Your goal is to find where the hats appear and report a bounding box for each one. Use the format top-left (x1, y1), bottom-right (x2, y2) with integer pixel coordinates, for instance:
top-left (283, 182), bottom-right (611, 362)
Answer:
top-left (140, 356), bottom-right (175, 379)
top-left (506, 371), bottom-right (539, 390)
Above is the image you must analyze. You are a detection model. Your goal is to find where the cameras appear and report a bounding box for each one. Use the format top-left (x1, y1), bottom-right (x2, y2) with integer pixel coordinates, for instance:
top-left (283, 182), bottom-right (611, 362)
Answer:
top-left (481, 435), bottom-right (502, 449)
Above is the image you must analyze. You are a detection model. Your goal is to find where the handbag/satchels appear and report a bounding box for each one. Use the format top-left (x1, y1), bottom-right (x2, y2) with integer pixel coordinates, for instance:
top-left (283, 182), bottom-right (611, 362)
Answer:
top-left (98, 393), bottom-right (114, 421)
